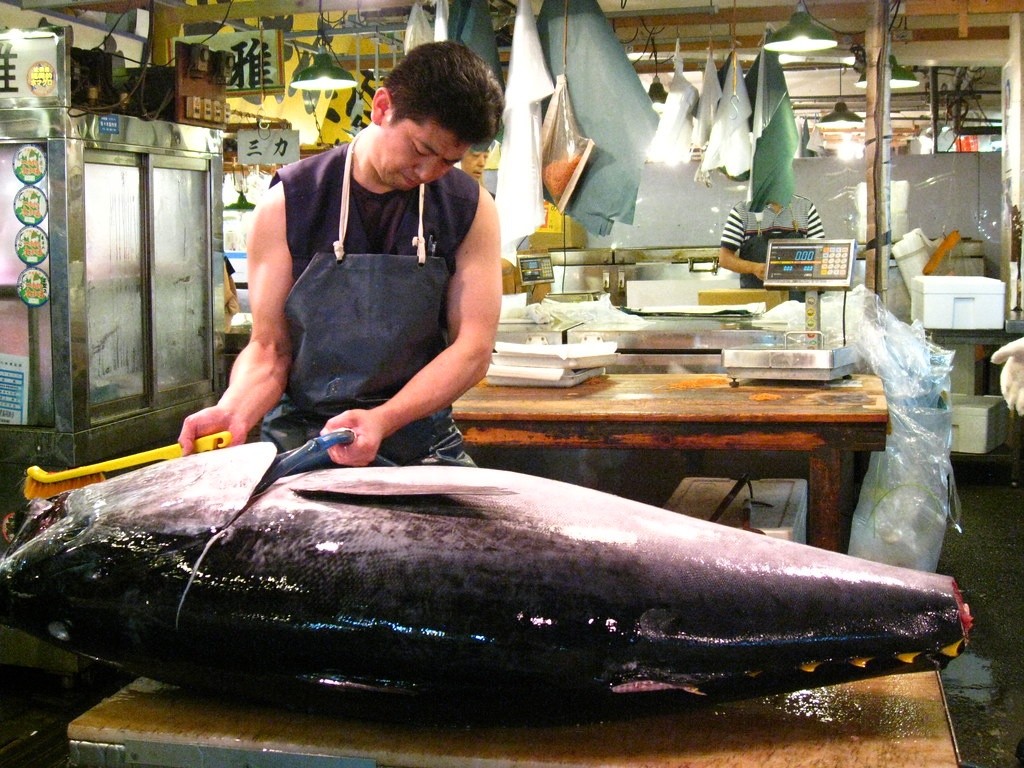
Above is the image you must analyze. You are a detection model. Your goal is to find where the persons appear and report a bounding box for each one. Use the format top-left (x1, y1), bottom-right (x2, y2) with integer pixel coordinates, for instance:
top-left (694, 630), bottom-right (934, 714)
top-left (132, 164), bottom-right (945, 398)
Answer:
top-left (989, 338), bottom-right (1024, 420)
top-left (462, 144), bottom-right (496, 200)
top-left (718, 193), bottom-right (825, 303)
top-left (176, 40), bottom-right (502, 476)
top-left (223, 255), bottom-right (239, 336)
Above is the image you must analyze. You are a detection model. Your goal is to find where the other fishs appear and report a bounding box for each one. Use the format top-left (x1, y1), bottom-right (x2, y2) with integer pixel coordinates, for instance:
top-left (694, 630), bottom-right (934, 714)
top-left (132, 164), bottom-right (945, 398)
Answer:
top-left (0, 440), bottom-right (972, 737)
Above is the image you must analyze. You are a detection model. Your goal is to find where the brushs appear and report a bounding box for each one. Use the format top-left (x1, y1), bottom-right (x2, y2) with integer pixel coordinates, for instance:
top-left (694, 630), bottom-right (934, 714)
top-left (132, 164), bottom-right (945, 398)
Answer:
top-left (23, 430), bottom-right (233, 501)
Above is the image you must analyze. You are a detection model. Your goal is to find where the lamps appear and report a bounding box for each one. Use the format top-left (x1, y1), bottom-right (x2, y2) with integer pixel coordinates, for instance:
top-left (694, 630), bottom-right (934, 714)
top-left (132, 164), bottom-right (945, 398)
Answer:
top-left (761, 3), bottom-right (837, 52)
top-left (851, 50), bottom-right (921, 89)
top-left (816, 68), bottom-right (862, 128)
top-left (289, 11), bottom-right (356, 90)
top-left (647, 39), bottom-right (667, 106)
top-left (224, 183), bottom-right (255, 212)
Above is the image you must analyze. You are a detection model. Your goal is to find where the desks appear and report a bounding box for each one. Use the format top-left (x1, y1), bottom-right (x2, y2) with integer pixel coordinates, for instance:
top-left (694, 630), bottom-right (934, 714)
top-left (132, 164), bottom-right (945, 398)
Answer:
top-left (64, 672), bottom-right (959, 768)
top-left (452, 371), bottom-right (889, 554)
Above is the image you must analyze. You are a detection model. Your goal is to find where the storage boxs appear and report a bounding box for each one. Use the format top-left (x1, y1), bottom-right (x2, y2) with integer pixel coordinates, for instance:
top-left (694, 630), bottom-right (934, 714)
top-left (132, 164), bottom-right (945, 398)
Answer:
top-left (947, 394), bottom-right (1007, 454)
top-left (910, 275), bottom-right (1007, 331)
top-left (663, 475), bottom-right (807, 544)
top-left (697, 289), bottom-right (792, 314)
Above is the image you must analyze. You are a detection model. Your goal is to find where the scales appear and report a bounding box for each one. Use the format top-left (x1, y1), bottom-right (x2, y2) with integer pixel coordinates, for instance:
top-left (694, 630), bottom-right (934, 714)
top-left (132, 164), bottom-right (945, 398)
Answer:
top-left (516, 253), bottom-right (601, 303)
top-left (720, 239), bottom-right (860, 387)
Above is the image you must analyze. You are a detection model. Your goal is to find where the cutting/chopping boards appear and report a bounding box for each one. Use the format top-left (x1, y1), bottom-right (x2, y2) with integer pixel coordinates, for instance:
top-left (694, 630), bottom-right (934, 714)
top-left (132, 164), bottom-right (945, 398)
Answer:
top-left (66, 671), bottom-right (961, 768)
